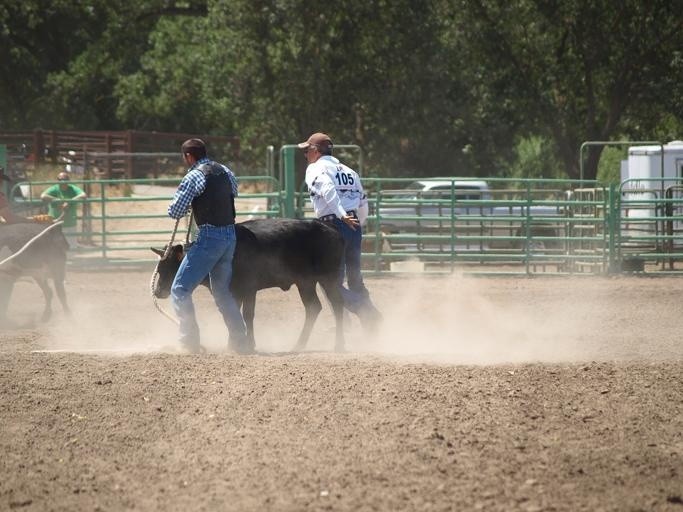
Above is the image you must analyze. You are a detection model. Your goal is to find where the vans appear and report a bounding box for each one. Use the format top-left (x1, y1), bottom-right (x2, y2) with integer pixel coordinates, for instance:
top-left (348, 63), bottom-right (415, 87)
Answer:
top-left (93, 139), bottom-right (153, 181)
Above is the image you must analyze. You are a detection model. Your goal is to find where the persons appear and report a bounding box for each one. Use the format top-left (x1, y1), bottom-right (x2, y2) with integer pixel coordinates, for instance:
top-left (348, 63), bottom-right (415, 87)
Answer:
top-left (39, 171), bottom-right (87, 270)
top-left (167, 137), bottom-right (247, 354)
top-left (0, 143), bottom-right (102, 224)
top-left (297, 133), bottom-right (385, 345)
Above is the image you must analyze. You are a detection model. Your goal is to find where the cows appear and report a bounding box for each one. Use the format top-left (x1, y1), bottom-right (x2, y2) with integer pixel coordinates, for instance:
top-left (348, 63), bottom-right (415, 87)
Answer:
top-left (0, 222), bottom-right (70, 324)
top-left (361, 228), bottom-right (392, 270)
top-left (150, 218), bottom-right (345, 353)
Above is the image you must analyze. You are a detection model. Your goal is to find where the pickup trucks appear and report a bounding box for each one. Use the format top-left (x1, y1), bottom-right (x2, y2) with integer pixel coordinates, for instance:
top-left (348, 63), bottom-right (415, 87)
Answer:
top-left (369, 176), bottom-right (567, 265)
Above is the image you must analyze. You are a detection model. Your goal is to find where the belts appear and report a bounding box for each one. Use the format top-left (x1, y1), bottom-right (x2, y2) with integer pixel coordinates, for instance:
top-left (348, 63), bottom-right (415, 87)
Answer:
top-left (320, 211), bottom-right (354, 220)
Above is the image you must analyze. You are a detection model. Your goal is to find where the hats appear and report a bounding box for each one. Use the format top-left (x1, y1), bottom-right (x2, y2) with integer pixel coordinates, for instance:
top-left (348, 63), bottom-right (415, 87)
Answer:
top-left (298, 132), bottom-right (333, 149)
